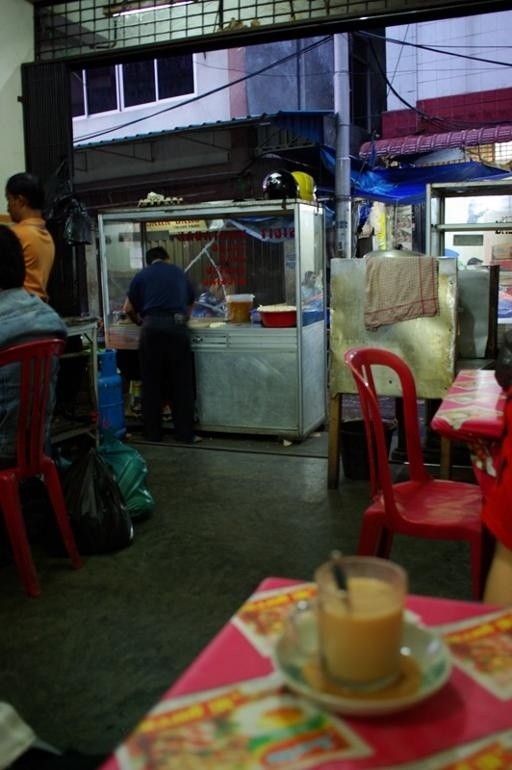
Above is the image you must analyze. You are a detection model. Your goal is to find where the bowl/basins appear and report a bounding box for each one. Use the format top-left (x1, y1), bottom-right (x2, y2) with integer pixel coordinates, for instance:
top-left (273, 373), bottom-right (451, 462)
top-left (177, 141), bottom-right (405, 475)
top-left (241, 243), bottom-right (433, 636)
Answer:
top-left (112, 311), bottom-right (132, 324)
top-left (258, 304), bottom-right (297, 327)
top-left (302, 299), bottom-right (323, 324)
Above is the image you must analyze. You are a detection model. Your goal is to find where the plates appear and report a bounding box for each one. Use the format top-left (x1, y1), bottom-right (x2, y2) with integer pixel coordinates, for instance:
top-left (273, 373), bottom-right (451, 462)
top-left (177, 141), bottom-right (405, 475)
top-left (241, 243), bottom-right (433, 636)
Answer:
top-left (272, 612), bottom-right (452, 712)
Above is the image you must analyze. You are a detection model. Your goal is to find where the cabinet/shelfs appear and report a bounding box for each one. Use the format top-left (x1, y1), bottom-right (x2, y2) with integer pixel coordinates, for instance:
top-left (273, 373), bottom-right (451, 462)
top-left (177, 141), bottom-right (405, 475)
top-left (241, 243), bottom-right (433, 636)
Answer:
top-left (49, 321), bottom-right (99, 461)
top-left (97, 198), bottom-right (326, 443)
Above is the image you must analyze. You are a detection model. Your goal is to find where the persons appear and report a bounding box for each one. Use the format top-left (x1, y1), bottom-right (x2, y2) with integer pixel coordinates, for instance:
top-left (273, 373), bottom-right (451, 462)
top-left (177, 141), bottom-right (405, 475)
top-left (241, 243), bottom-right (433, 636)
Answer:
top-left (6, 173), bottom-right (55, 304)
top-left (300, 271), bottom-right (323, 304)
top-left (1, 225), bottom-right (68, 471)
top-left (123, 245), bottom-right (204, 445)
top-left (208, 262), bottom-right (235, 300)
top-left (478, 430), bottom-right (512, 604)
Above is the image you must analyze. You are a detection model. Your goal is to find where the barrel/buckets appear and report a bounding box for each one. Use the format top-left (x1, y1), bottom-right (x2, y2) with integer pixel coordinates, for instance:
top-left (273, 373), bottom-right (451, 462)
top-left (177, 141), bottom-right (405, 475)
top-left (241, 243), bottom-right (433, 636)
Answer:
top-left (225, 294), bottom-right (255, 322)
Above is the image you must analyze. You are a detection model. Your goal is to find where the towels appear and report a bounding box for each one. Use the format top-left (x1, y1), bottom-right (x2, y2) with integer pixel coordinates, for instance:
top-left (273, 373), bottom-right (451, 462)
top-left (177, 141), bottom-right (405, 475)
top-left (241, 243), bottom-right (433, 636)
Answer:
top-left (364, 256), bottom-right (439, 330)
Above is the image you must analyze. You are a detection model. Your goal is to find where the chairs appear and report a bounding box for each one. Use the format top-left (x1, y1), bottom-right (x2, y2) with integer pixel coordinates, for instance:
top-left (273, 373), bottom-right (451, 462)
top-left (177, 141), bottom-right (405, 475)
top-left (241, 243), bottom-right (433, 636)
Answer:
top-left (344, 348), bottom-right (489, 602)
top-left (0, 338), bottom-right (82, 597)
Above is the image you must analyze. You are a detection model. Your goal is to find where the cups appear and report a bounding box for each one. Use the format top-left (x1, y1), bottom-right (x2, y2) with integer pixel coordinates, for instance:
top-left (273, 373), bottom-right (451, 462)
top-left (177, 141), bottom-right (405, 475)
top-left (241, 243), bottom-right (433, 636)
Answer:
top-left (313, 555), bottom-right (409, 693)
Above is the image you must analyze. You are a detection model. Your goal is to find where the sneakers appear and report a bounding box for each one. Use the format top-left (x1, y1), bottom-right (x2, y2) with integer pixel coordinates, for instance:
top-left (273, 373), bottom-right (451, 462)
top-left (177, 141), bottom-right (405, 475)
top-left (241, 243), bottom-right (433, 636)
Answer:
top-left (146, 433), bottom-right (169, 442)
top-left (177, 434), bottom-right (203, 444)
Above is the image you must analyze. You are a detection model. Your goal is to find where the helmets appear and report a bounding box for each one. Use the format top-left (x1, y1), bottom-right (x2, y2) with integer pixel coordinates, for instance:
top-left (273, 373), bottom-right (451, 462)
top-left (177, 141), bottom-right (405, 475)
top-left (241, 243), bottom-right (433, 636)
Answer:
top-left (288, 169), bottom-right (318, 201)
top-left (259, 168), bottom-right (298, 200)
